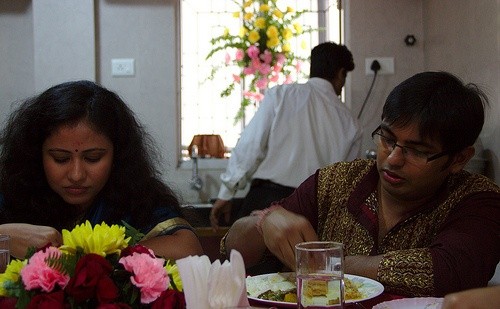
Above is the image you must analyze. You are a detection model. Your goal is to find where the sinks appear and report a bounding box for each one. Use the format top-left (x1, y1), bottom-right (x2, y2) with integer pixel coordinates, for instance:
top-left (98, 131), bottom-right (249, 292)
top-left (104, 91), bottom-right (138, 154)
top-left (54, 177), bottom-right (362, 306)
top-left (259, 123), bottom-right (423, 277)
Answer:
top-left (180, 207), bottom-right (230, 228)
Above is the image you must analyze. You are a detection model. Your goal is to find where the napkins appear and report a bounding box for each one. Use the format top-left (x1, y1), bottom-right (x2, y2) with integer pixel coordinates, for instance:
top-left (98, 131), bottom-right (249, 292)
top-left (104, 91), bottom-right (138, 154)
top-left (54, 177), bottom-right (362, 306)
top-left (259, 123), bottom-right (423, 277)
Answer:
top-left (175, 248), bottom-right (251, 309)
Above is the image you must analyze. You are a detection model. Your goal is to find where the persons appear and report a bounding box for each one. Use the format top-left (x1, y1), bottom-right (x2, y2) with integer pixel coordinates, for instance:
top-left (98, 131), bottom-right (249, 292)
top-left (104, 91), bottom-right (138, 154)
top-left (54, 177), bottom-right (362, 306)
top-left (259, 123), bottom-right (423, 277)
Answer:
top-left (0, 80), bottom-right (206, 265)
top-left (220, 71), bottom-right (500, 309)
top-left (210, 41), bottom-right (364, 234)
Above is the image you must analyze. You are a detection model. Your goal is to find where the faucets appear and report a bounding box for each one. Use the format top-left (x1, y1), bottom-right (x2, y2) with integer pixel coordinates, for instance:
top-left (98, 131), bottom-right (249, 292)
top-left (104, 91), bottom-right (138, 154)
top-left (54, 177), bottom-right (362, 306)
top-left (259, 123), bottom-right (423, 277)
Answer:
top-left (190, 144), bottom-right (202, 191)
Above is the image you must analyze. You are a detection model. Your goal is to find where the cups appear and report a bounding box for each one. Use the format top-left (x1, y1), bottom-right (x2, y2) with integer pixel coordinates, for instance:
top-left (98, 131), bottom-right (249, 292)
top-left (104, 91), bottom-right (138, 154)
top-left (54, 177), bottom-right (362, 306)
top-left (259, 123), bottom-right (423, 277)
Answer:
top-left (0, 234), bottom-right (10, 274)
top-left (294, 241), bottom-right (345, 309)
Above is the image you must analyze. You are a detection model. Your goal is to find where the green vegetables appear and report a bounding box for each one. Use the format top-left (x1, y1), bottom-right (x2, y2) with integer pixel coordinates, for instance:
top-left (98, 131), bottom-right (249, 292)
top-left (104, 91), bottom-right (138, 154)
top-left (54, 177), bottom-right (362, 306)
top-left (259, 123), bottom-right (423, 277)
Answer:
top-left (258, 288), bottom-right (298, 302)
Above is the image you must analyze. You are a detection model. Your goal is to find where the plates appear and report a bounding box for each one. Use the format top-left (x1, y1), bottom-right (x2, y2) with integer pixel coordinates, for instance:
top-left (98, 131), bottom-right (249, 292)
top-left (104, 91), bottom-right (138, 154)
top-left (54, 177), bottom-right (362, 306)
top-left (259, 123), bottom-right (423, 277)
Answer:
top-left (372, 297), bottom-right (444, 309)
top-left (245, 272), bottom-right (385, 309)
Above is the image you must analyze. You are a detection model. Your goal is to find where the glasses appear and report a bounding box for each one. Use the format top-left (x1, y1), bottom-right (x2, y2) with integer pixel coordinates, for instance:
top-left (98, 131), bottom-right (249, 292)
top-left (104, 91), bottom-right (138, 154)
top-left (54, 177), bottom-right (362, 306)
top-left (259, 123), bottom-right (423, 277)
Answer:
top-left (372, 124), bottom-right (446, 168)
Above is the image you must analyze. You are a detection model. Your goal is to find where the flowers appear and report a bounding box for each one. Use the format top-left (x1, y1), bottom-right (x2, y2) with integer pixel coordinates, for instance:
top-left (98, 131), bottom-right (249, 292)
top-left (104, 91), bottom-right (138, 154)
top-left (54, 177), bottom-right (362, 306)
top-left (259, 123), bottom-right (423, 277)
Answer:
top-left (0, 218), bottom-right (184, 309)
top-left (206, 0), bottom-right (327, 126)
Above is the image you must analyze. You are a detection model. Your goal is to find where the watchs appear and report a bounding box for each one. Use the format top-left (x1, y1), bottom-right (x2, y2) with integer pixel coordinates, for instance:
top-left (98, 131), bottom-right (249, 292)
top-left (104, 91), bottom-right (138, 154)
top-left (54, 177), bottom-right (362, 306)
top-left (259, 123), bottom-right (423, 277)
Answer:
top-left (334, 256), bottom-right (345, 271)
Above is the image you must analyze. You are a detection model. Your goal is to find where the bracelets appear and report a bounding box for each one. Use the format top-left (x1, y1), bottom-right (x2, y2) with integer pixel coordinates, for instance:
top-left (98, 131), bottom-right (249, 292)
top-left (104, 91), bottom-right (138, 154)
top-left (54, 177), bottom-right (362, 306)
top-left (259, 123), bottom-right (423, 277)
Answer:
top-left (257, 205), bottom-right (283, 233)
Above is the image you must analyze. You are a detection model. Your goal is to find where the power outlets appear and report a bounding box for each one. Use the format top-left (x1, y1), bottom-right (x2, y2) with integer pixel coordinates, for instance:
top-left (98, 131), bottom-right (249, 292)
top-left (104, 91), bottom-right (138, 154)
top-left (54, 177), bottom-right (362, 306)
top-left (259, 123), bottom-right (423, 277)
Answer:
top-left (364, 56), bottom-right (395, 76)
top-left (111, 58), bottom-right (135, 76)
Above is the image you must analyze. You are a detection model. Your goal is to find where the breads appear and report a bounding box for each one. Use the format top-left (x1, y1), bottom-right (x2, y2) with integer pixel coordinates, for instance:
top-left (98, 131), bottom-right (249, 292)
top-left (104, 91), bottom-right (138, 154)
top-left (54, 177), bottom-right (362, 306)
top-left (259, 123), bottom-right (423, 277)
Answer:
top-left (302, 274), bottom-right (360, 300)
